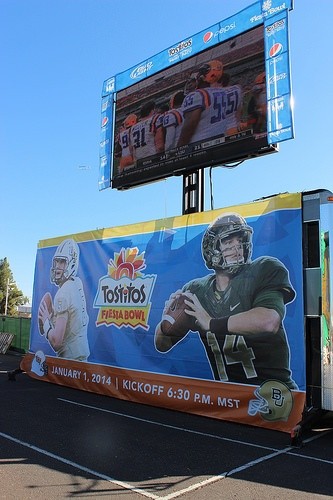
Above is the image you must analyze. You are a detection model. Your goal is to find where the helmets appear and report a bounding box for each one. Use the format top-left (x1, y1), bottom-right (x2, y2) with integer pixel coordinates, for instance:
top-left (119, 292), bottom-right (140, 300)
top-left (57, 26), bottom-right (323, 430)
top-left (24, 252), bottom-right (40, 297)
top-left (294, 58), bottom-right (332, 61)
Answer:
top-left (50, 239), bottom-right (80, 285)
top-left (200, 212), bottom-right (253, 270)
top-left (170, 90), bottom-right (187, 110)
top-left (254, 71), bottom-right (265, 89)
top-left (139, 99), bottom-right (156, 120)
top-left (124, 114), bottom-right (137, 130)
top-left (183, 76), bottom-right (197, 95)
top-left (194, 60), bottom-right (223, 87)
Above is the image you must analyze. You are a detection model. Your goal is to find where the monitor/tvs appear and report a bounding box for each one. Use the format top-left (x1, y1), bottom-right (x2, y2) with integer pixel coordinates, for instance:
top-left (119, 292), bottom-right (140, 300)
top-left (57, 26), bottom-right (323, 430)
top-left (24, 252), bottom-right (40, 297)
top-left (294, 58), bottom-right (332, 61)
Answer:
top-left (110, 22), bottom-right (280, 193)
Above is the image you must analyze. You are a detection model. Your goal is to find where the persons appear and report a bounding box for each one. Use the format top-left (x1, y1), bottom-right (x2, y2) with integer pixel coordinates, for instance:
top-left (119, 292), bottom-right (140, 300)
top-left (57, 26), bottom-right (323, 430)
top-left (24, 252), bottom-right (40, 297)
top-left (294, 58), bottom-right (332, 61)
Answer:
top-left (152, 212), bottom-right (301, 394)
top-left (37, 238), bottom-right (91, 361)
top-left (114, 60), bottom-right (266, 168)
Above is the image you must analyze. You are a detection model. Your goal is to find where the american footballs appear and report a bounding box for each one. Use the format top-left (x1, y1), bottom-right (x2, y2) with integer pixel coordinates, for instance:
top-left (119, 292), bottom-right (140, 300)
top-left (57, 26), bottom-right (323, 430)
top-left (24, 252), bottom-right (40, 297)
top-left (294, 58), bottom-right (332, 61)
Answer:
top-left (161, 291), bottom-right (195, 338)
top-left (38, 293), bottom-right (53, 335)
